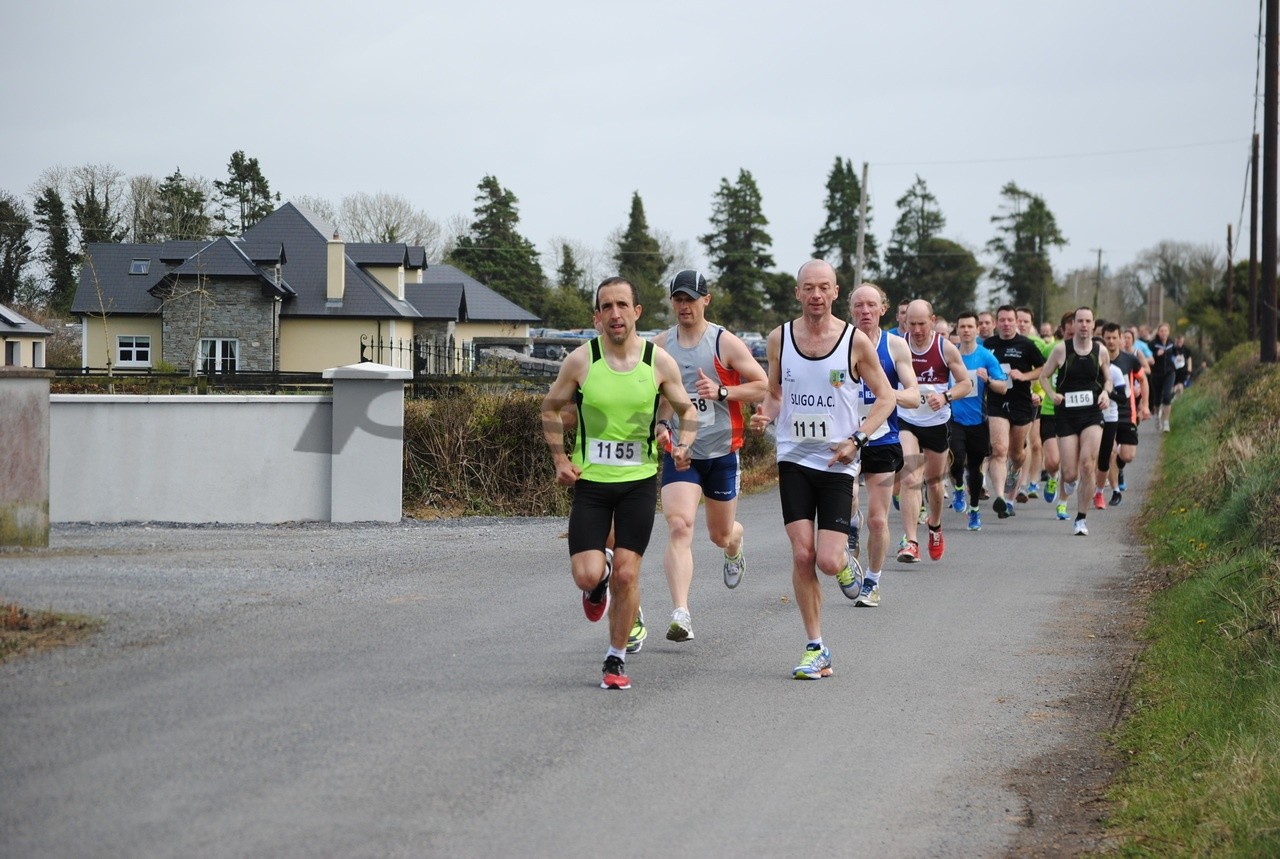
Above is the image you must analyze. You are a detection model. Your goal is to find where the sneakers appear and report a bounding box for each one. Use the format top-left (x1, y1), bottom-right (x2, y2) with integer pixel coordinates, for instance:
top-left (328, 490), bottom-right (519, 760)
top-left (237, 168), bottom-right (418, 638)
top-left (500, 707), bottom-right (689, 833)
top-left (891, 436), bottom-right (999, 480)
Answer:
top-left (1044, 471), bottom-right (1059, 503)
top-left (1005, 462), bottom-right (1020, 493)
top-left (1016, 486), bottom-right (1029, 504)
top-left (979, 487), bottom-right (990, 500)
top-left (600, 655), bottom-right (631, 689)
top-left (897, 535), bottom-right (908, 551)
top-left (835, 543), bottom-right (864, 600)
top-left (583, 548), bottom-right (615, 622)
top-left (966, 508), bottom-right (982, 531)
top-left (1056, 468), bottom-right (1125, 535)
top-left (897, 541), bottom-right (921, 563)
top-left (953, 482), bottom-right (967, 513)
top-left (723, 537), bottom-right (746, 590)
top-left (928, 518), bottom-right (944, 561)
top-left (847, 510), bottom-right (864, 559)
top-left (665, 607), bottom-right (694, 642)
top-left (792, 642), bottom-right (833, 680)
top-left (627, 607), bottom-right (648, 654)
top-left (1027, 482), bottom-right (1039, 499)
top-left (858, 473), bottom-right (949, 526)
top-left (854, 578), bottom-right (881, 607)
top-left (992, 496), bottom-right (1016, 519)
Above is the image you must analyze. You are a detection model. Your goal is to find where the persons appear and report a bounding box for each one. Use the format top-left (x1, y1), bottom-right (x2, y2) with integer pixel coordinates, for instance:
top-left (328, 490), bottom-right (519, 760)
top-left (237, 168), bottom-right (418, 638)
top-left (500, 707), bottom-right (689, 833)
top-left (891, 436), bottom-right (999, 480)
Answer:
top-left (749, 259), bottom-right (899, 681)
top-left (541, 277), bottom-right (699, 690)
top-left (844, 281), bottom-right (921, 607)
top-left (885, 298), bottom-right (1194, 517)
top-left (895, 299), bottom-right (972, 563)
top-left (1038, 306), bottom-right (1120, 537)
top-left (946, 310), bottom-right (1008, 530)
top-left (648, 271), bottom-right (769, 642)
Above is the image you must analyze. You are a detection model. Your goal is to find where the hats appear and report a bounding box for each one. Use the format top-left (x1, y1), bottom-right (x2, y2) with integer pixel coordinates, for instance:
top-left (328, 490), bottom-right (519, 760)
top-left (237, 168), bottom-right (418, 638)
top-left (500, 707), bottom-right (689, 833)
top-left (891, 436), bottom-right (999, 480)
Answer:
top-left (669, 270), bottom-right (707, 301)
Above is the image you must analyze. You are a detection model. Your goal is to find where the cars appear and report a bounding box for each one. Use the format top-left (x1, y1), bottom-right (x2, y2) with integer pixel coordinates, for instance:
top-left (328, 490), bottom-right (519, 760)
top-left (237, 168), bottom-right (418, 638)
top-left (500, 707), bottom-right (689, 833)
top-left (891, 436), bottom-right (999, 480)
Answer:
top-left (634, 329), bottom-right (668, 342)
top-left (530, 327), bottom-right (600, 339)
top-left (737, 332), bottom-right (762, 346)
top-left (750, 340), bottom-right (768, 360)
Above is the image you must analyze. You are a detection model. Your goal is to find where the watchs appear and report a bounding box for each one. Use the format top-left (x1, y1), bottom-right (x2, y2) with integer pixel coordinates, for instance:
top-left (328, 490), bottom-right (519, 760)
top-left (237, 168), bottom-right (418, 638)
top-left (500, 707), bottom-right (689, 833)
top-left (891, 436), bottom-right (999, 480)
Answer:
top-left (718, 385), bottom-right (728, 401)
top-left (852, 429), bottom-right (869, 448)
top-left (1103, 389), bottom-right (1113, 398)
top-left (676, 443), bottom-right (694, 453)
top-left (942, 390), bottom-right (953, 403)
top-left (657, 419), bottom-right (673, 432)
top-left (984, 376), bottom-right (992, 385)
top-left (846, 431), bottom-right (866, 450)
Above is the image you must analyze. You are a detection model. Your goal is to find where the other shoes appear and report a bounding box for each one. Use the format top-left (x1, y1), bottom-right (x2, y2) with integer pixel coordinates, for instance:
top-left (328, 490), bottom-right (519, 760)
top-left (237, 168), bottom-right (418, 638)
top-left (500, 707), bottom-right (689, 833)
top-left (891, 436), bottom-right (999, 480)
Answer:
top-left (1154, 416), bottom-right (1170, 433)
